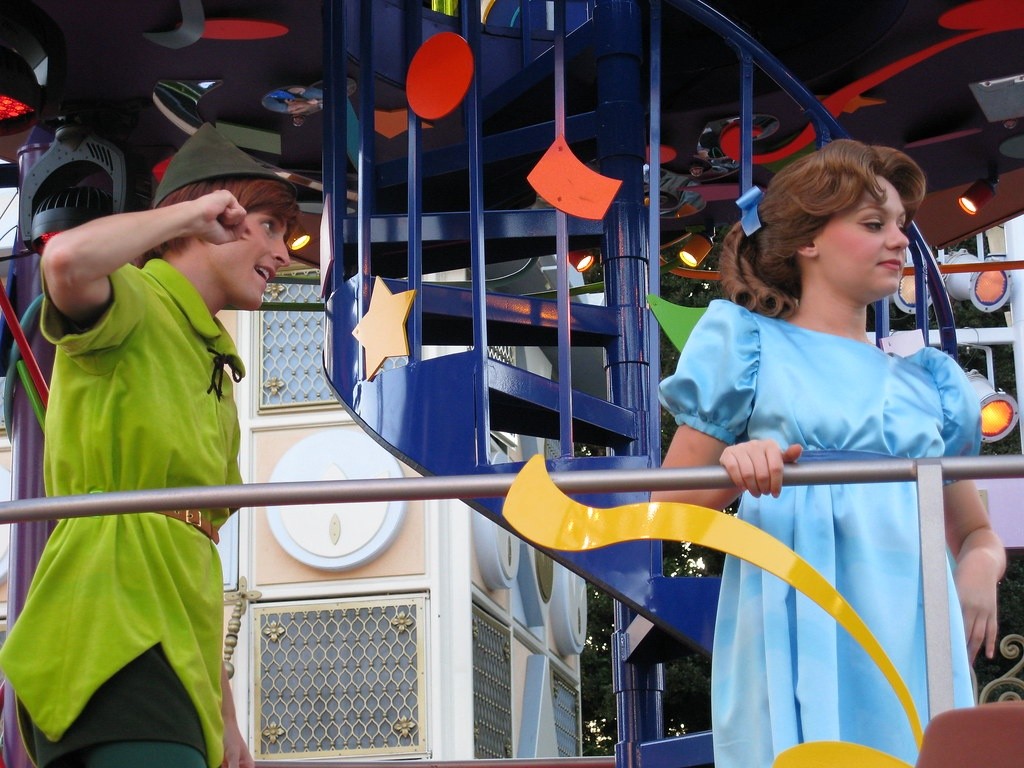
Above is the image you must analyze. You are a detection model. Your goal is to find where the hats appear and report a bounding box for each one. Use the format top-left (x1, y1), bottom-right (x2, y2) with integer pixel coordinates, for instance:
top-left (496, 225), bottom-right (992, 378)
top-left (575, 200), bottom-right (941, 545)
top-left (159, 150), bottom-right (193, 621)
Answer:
top-left (154, 122), bottom-right (296, 206)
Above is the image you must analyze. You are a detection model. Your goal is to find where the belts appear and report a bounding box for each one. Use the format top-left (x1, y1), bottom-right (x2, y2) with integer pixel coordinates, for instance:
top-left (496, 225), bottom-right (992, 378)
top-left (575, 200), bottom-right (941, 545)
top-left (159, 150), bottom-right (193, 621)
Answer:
top-left (158, 505), bottom-right (220, 545)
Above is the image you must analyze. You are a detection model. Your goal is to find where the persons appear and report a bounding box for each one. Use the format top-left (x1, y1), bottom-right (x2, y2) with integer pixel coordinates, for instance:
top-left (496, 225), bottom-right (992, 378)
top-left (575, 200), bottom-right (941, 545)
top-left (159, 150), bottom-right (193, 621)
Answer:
top-left (648, 139), bottom-right (1006, 768)
top-left (15, 122), bottom-right (300, 768)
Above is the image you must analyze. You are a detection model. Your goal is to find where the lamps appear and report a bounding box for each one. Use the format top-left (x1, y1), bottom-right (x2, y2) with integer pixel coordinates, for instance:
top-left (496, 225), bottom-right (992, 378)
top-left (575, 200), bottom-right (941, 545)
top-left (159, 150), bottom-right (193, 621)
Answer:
top-left (680, 226), bottom-right (718, 268)
top-left (962, 365), bottom-right (1018, 443)
top-left (936, 248), bottom-right (1010, 312)
top-left (286, 224), bottom-right (311, 251)
top-left (890, 262), bottom-right (934, 314)
top-left (569, 249), bottom-right (596, 274)
top-left (958, 175), bottom-right (1001, 214)
top-left (21, 121), bottom-right (127, 256)
